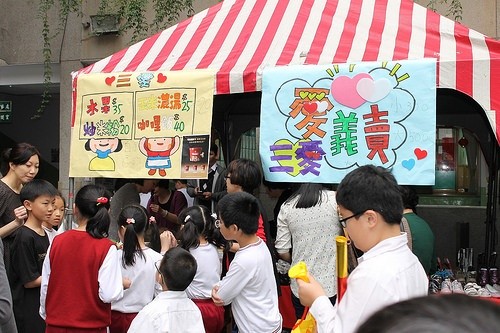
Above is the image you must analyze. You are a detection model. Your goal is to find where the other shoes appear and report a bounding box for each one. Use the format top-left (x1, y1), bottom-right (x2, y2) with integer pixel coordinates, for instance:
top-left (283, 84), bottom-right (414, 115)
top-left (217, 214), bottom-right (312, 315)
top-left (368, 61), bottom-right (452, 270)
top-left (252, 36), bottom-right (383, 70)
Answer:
top-left (454, 268), bottom-right (465, 281)
top-left (493, 282), bottom-right (500, 291)
top-left (464, 283), bottom-right (481, 296)
top-left (478, 288), bottom-right (491, 297)
top-left (485, 283), bottom-right (500, 297)
top-left (429, 275), bottom-right (441, 293)
top-left (466, 270), bottom-right (477, 282)
top-left (434, 270), bottom-right (453, 279)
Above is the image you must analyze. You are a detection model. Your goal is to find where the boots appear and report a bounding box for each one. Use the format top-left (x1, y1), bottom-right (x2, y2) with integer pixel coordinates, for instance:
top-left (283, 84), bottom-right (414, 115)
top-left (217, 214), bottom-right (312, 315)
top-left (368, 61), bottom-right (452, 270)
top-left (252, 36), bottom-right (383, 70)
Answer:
top-left (490, 268), bottom-right (500, 285)
top-left (458, 248), bottom-right (466, 271)
top-left (477, 268), bottom-right (488, 285)
top-left (464, 247), bottom-right (473, 271)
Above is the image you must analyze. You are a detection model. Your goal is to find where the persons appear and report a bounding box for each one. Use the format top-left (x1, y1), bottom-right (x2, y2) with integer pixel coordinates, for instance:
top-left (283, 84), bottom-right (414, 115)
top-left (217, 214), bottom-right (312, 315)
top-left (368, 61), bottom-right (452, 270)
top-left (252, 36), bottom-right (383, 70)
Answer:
top-left (157, 206), bottom-right (226, 332)
top-left (219, 158), bottom-right (271, 259)
top-left (0, 142), bottom-right (41, 265)
top-left (109, 178), bottom-right (160, 241)
top-left (39, 184), bottom-right (132, 333)
top-left (7, 179), bottom-right (58, 333)
top-left (274, 182), bottom-right (354, 333)
top-left (397, 184), bottom-right (435, 277)
top-left (0, 237), bottom-right (18, 333)
top-left (355, 291), bottom-right (500, 332)
top-left (41, 191), bottom-right (65, 243)
top-left (108, 203), bottom-right (165, 333)
top-left (295, 164), bottom-right (429, 333)
top-left (211, 192), bottom-right (283, 333)
top-left (144, 180), bottom-right (187, 226)
top-left (186, 142), bottom-right (228, 209)
top-left (126, 247), bottom-right (207, 333)
top-left (173, 178), bottom-right (195, 208)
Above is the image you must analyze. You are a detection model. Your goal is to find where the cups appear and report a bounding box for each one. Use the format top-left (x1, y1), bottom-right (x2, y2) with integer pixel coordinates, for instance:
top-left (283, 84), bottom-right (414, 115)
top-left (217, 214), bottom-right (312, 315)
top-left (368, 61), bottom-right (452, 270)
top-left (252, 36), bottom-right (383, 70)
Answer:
top-left (458, 248), bottom-right (473, 273)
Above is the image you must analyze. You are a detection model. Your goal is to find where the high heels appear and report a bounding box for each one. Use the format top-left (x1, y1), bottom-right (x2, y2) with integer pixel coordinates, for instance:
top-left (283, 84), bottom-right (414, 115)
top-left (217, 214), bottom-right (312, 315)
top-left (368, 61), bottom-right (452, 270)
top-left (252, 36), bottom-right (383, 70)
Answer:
top-left (437, 255), bottom-right (443, 271)
top-left (442, 255), bottom-right (451, 270)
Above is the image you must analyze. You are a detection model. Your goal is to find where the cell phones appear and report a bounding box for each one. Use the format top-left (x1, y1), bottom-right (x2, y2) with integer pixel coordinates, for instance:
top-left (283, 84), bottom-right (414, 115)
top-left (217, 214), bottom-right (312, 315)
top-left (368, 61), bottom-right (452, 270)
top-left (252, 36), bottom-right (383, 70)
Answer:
top-left (197, 191), bottom-right (203, 194)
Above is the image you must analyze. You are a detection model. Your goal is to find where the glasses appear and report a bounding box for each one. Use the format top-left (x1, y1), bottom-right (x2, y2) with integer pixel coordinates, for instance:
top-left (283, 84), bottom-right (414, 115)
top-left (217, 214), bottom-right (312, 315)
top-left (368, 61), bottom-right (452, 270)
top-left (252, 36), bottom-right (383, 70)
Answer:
top-left (338, 208), bottom-right (374, 228)
top-left (154, 260), bottom-right (164, 284)
top-left (223, 174), bottom-right (230, 180)
top-left (215, 219), bottom-right (226, 228)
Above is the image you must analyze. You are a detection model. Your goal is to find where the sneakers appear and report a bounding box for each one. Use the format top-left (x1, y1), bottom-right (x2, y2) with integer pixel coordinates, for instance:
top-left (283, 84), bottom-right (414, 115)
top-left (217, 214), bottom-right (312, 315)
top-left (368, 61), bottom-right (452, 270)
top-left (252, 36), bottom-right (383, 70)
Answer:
top-left (452, 280), bottom-right (463, 293)
top-left (441, 279), bottom-right (451, 293)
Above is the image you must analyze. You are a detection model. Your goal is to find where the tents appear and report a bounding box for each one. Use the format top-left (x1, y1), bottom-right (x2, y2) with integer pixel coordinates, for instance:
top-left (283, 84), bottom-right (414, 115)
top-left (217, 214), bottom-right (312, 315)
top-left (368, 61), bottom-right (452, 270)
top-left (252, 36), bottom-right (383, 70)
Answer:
top-left (67, 0), bottom-right (496, 301)
